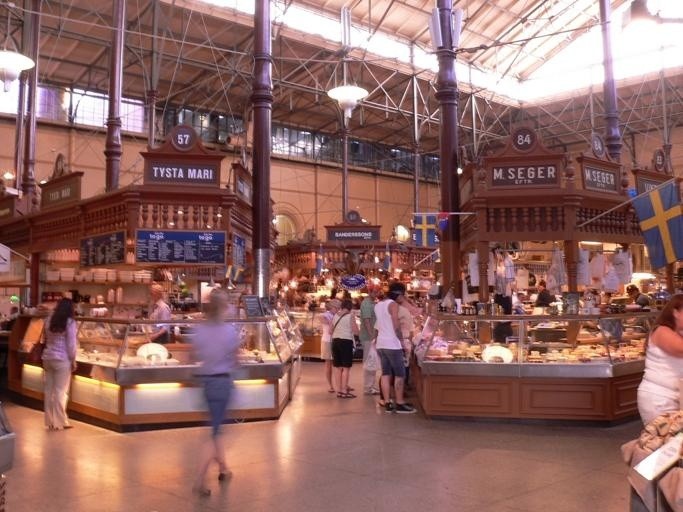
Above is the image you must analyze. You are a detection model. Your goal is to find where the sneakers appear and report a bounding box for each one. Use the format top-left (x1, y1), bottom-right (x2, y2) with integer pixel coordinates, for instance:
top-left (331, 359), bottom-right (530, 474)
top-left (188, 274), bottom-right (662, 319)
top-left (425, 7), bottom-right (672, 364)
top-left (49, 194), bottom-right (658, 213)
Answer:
top-left (395, 401), bottom-right (419, 415)
top-left (384, 400), bottom-right (395, 414)
top-left (363, 386), bottom-right (380, 396)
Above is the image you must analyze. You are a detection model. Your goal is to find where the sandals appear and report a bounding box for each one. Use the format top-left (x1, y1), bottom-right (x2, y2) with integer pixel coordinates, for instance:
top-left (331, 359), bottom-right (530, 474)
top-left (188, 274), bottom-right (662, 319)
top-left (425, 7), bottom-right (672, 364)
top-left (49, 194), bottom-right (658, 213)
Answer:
top-left (341, 391), bottom-right (357, 399)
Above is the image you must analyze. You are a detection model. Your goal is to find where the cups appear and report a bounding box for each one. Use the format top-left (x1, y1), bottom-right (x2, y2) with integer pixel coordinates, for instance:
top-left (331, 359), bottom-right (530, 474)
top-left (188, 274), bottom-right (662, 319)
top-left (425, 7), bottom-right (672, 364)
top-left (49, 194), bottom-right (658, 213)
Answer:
top-left (579, 300), bottom-right (601, 316)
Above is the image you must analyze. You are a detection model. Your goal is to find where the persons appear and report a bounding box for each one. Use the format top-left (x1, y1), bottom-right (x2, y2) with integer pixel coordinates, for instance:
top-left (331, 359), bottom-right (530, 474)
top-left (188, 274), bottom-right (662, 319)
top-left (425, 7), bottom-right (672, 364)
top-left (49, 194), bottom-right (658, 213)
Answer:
top-left (41, 297), bottom-right (77, 430)
top-left (511, 281), bottom-right (556, 317)
top-left (626, 284), bottom-right (649, 333)
top-left (148, 285), bottom-right (172, 342)
top-left (5, 305), bottom-right (20, 319)
top-left (320, 283), bottom-right (426, 415)
top-left (635, 295), bottom-right (683, 429)
top-left (189, 290), bottom-right (241, 495)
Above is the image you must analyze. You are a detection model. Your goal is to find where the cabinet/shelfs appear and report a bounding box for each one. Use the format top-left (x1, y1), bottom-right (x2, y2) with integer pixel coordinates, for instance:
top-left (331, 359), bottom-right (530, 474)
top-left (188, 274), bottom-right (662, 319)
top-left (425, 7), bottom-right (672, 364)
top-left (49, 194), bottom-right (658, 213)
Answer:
top-left (38, 253), bottom-right (201, 315)
top-left (409, 305), bottom-right (664, 427)
top-left (513, 258), bottom-right (595, 303)
top-left (299, 309), bottom-right (363, 361)
top-left (5, 309), bottom-right (296, 432)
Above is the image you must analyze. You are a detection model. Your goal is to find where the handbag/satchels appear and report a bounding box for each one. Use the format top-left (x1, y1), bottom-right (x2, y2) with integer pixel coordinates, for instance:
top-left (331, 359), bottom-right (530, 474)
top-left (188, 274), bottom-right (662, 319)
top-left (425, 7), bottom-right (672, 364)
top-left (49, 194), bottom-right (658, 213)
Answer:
top-left (27, 342), bottom-right (46, 370)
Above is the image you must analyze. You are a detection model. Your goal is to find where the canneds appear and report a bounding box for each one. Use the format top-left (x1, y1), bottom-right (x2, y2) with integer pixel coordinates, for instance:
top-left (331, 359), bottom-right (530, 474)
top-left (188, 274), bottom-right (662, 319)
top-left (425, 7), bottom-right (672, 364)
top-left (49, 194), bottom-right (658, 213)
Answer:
top-left (460, 305), bottom-right (477, 316)
top-left (606, 304), bottom-right (627, 314)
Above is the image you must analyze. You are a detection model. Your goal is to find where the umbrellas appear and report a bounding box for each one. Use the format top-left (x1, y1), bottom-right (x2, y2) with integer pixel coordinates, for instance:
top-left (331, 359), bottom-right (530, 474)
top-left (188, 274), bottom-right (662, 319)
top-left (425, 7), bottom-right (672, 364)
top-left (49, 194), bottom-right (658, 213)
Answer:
top-left (340, 273), bottom-right (367, 291)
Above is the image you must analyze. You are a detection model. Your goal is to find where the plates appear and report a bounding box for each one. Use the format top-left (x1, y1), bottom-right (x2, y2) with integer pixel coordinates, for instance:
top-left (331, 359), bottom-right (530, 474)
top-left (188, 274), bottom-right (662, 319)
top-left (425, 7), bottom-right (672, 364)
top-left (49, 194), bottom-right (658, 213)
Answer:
top-left (44, 265), bottom-right (152, 284)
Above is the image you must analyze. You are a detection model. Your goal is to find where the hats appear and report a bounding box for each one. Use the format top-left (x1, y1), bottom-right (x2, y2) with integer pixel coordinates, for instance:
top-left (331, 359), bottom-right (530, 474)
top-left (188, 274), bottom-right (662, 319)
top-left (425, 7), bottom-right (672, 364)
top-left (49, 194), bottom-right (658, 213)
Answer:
top-left (386, 282), bottom-right (405, 296)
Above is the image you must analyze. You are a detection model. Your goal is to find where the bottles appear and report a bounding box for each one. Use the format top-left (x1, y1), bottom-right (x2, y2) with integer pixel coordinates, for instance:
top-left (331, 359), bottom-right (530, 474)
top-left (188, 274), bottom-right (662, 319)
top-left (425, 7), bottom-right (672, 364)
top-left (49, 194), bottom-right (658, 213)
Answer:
top-left (107, 286), bottom-right (123, 304)
top-left (45, 248), bottom-right (80, 261)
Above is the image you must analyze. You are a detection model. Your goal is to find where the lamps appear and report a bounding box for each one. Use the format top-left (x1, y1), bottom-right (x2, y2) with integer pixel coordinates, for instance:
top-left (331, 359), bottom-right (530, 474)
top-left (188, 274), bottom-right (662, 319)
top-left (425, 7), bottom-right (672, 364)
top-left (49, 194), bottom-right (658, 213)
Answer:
top-left (0, 0), bottom-right (35, 93)
top-left (325, 0), bottom-right (368, 130)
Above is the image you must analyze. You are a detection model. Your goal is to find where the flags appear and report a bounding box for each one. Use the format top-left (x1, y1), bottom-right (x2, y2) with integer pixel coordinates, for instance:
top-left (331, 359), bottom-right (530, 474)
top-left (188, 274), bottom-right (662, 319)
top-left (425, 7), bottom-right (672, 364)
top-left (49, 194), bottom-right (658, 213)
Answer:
top-left (413, 213), bottom-right (437, 246)
top-left (632, 181), bottom-right (683, 268)
